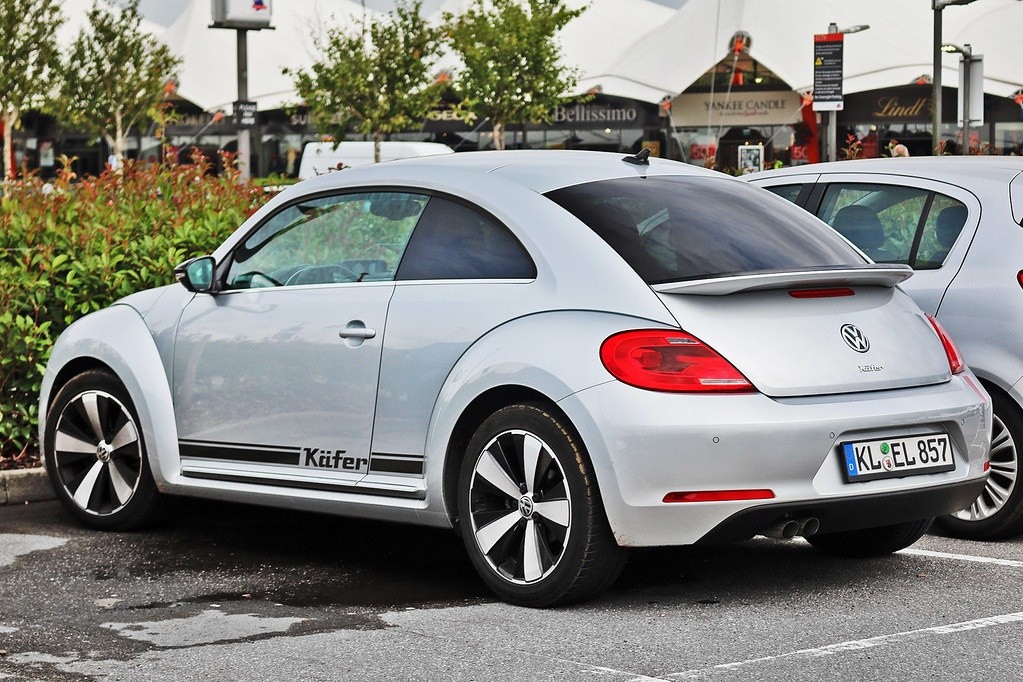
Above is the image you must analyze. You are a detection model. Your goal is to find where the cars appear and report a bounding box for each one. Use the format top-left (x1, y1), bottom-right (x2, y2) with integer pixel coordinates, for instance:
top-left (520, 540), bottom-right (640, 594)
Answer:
top-left (38, 150), bottom-right (994, 610)
top-left (634, 155), bottom-right (1023, 541)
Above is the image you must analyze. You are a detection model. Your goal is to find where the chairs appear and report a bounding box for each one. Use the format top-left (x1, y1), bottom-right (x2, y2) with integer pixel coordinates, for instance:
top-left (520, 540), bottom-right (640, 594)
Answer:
top-left (833, 204), bottom-right (897, 261)
top-left (929, 205), bottom-right (968, 268)
top-left (597, 206), bottom-right (679, 284)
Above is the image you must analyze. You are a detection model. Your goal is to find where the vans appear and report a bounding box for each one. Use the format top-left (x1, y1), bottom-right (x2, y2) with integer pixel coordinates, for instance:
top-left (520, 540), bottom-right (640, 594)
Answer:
top-left (299, 141), bottom-right (454, 183)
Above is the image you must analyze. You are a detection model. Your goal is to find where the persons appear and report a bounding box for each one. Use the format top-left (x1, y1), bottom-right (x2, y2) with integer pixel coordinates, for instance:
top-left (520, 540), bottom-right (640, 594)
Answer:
top-left (893, 144), bottom-right (909, 157)
top-left (107, 147), bottom-right (114, 165)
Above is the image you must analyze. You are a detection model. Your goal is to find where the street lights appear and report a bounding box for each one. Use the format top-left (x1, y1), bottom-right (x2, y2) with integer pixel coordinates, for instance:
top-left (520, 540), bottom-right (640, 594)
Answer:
top-left (931, 0), bottom-right (977, 155)
top-left (828, 23), bottom-right (870, 162)
top-left (940, 42), bottom-right (974, 156)
